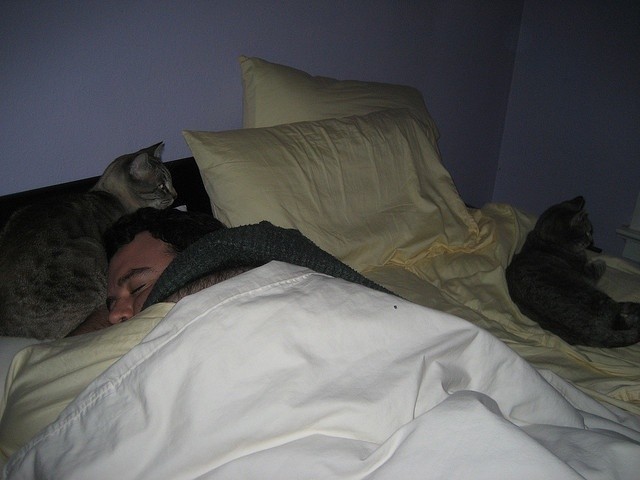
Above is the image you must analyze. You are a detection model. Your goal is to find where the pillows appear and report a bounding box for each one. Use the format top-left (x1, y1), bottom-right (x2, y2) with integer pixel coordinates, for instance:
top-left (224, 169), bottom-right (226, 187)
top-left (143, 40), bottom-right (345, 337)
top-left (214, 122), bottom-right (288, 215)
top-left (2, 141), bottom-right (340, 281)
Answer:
top-left (180, 106), bottom-right (480, 273)
top-left (237, 53), bottom-right (441, 141)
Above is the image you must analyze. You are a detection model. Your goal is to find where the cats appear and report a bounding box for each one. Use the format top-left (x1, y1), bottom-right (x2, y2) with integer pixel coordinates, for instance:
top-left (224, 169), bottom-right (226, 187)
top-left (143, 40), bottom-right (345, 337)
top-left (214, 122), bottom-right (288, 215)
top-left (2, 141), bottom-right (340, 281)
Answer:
top-left (1, 139), bottom-right (179, 341)
top-left (506, 196), bottom-right (640, 348)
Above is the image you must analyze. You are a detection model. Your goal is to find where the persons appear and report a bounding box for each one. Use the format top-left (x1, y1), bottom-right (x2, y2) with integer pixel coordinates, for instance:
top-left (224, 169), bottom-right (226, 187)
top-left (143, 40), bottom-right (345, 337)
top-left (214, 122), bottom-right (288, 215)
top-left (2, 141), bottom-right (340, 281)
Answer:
top-left (97, 203), bottom-right (260, 325)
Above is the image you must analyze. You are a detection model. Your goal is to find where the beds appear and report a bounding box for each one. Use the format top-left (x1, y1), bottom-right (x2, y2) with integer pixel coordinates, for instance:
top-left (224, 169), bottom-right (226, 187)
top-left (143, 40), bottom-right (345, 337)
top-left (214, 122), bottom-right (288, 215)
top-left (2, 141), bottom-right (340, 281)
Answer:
top-left (1, 156), bottom-right (640, 480)
